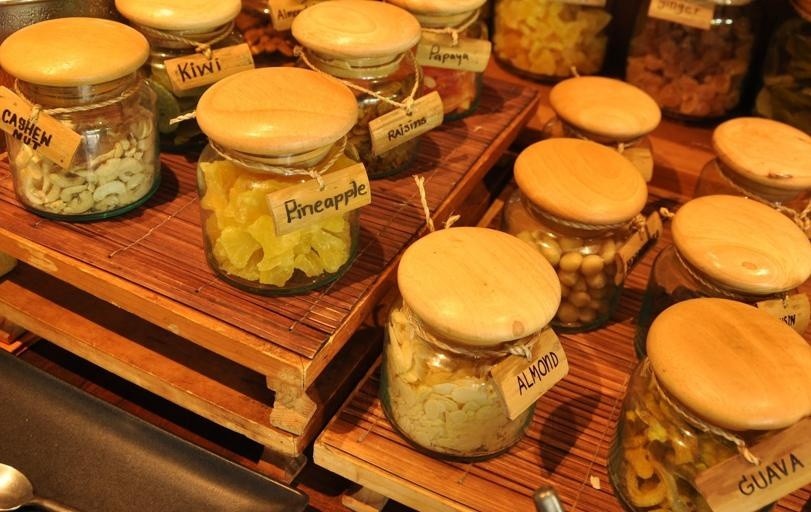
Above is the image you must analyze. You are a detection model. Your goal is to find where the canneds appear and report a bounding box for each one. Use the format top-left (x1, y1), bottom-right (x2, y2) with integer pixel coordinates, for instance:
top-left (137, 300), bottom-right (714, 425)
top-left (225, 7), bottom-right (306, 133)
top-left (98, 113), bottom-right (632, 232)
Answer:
top-left (381, 227), bottom-right (562, 461)
top-left (608, 296), bottom-right (811, 512)
top-left (503, 75), bottom-right (662, 331)
top-left (195, 66), bottom-right (373, 292)
top-left (691, 117), bottom-right (811, 231)
top-left (633, 194), bottom-right (811, 360)
top-left (0, 0), bottom-right (489, 224)
